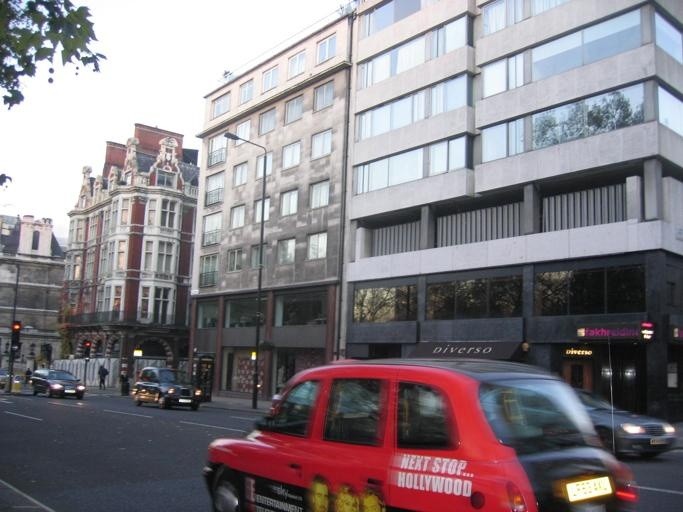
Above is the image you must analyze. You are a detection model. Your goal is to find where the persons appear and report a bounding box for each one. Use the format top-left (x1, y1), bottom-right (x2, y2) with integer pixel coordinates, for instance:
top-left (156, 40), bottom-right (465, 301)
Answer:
top-left (96, 365), bottom-right (108, 391)
top-left (23, 367), bottom-right (32, 386)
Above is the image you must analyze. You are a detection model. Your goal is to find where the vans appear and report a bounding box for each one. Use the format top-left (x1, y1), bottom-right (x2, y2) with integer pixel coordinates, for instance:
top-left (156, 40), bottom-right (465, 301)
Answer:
top-left (30, 367), bottom-right (85, 399)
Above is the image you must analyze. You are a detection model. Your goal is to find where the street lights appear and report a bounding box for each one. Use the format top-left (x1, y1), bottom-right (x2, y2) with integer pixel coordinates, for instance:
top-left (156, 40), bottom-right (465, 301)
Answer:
top-left (224, 132), bottom-right (267, 409)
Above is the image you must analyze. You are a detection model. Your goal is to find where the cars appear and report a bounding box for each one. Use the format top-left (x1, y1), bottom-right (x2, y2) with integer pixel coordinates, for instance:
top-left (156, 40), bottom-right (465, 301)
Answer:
top-left (200, 355), bottom-right (638, 511)
top-left (0, 367), bottom-right (25, 389)
top-left (479, 385), bottom-right (677, 462)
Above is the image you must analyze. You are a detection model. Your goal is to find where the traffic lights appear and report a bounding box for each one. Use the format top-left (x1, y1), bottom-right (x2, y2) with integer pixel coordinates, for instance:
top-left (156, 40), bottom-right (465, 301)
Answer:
top-left (11, 321), bottom-right (22, 350)
top-left (85, 339), bottom-right (91, 357)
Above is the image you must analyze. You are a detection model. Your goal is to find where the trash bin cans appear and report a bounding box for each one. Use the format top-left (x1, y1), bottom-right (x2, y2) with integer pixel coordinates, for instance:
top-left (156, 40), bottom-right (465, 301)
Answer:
top-left (121, 375), bottom-right (129, 396)
top-left (272, 385), bottom-right (285, 404)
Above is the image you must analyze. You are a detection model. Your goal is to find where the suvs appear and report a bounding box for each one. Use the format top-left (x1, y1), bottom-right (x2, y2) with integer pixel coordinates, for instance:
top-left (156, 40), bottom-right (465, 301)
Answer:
top-left (131, 365), bottom-right (203, 410)
